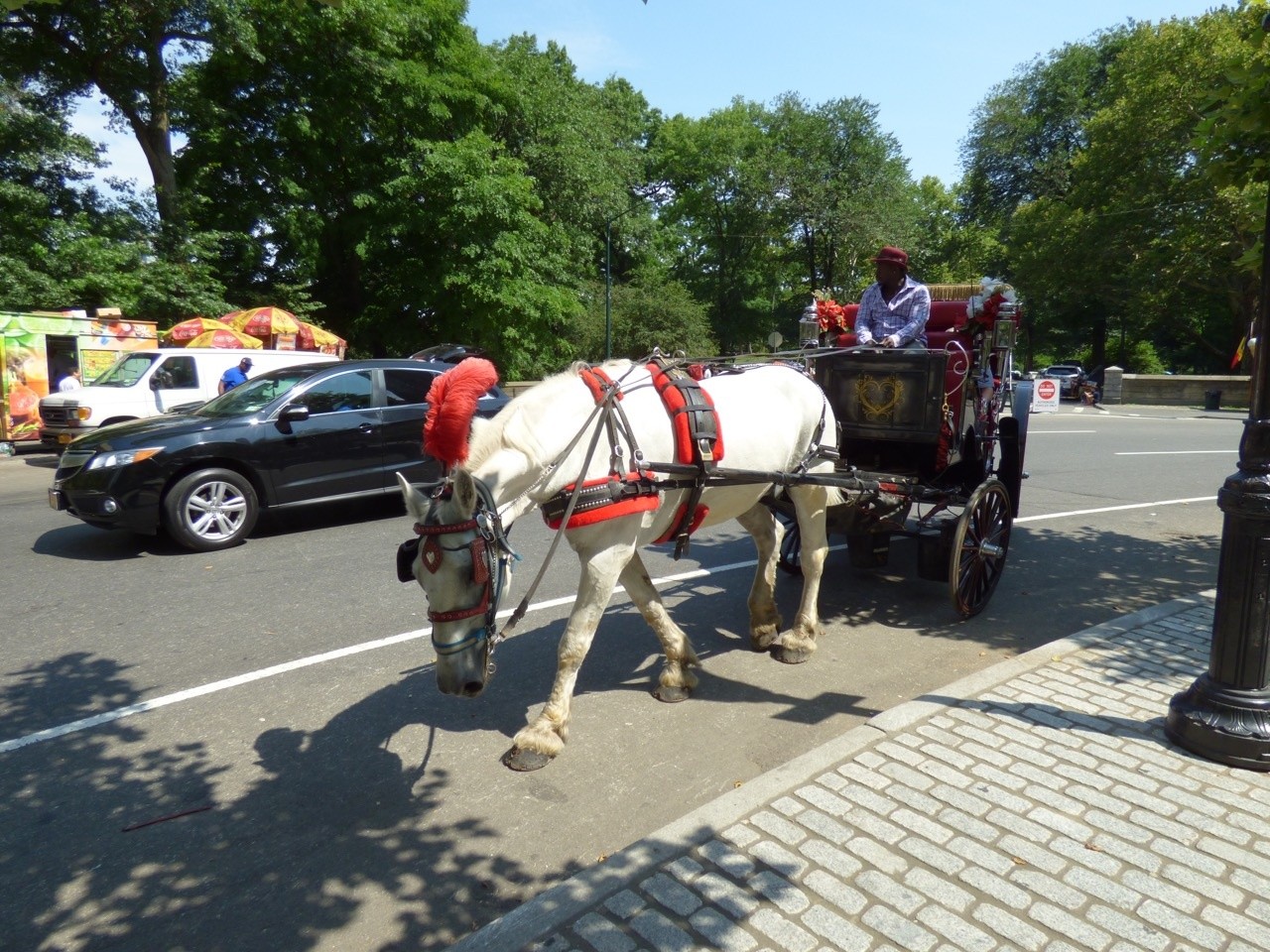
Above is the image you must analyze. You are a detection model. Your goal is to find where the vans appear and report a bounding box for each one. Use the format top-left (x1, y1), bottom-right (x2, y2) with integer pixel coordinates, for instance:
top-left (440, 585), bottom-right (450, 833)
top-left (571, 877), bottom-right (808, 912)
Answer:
top-left (37, 349), bottom-right (340, 456)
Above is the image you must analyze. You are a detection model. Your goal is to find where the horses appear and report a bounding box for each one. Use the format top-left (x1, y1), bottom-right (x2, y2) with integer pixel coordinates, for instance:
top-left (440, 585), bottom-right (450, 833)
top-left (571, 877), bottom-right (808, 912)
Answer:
top-left (395, 348), bottom-right (843, 773)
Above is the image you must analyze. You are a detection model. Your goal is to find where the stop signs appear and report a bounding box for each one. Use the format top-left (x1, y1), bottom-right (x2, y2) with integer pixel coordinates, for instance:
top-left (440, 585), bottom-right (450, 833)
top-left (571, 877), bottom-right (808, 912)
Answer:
top-left (1038, 381), bottom-right (1055, 399)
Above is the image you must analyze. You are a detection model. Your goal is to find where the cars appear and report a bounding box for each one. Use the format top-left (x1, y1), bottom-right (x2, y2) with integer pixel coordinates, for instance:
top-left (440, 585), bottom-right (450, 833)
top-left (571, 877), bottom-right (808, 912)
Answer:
top-left (1007, 370), bottom-right (1038, 380)
top-left (1036, 366), bottom-right (1084, 402)
top-left (49, 344), bottom-right (511, 553)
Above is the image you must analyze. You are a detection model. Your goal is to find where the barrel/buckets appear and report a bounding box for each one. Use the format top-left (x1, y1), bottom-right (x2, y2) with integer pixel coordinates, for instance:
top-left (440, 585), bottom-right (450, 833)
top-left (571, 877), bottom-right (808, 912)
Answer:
top-left (917, 539), bottom-right (955, 582)
top-left (848, 532), bottom-right (891, 568)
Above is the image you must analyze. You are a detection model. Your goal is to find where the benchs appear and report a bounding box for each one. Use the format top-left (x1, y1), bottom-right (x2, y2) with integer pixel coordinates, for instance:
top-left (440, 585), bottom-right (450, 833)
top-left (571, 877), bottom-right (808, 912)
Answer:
top-left (816, 301), bottom-right (979, 407)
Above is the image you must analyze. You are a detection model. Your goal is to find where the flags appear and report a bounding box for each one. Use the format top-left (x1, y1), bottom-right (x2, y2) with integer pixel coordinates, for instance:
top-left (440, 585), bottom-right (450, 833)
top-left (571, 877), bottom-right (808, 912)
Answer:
top-left (1231, 321), bottom-right (1252, 368)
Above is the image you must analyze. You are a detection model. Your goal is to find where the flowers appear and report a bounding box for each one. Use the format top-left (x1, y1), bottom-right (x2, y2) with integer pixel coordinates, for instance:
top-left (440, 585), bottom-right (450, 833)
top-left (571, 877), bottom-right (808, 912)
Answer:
top-left (966, 291), bottom-right (1023, 334)
top-left (811, 294), bottom-right (849, 333)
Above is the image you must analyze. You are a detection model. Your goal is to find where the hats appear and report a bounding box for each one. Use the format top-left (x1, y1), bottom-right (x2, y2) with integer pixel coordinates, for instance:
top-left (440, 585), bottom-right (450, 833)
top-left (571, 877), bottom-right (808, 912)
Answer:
top-left (241, 357), bottom-right (254, 366)
top-left (870, 247), bottom-right (909, 272)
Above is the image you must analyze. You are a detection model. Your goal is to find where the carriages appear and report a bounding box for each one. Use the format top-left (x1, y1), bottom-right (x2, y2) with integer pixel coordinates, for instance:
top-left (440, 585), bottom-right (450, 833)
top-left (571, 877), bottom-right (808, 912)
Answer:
top-left (392, 278), bottom-right (1034, 772)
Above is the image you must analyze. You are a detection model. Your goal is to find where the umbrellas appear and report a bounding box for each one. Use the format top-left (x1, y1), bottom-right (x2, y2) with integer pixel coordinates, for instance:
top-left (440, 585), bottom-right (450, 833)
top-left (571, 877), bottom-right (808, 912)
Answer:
top-left (161, 305), bottom-right (348, 349)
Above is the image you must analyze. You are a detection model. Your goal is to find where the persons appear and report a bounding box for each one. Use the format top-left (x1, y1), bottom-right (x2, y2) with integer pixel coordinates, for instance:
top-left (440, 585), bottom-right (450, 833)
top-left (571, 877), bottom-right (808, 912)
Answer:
top-left (218, 358), bottom-right (253, 395)
top-left (47, 346), bottom-right (82, 394)
top-left (853, 245), bottom-right (932, 357)
